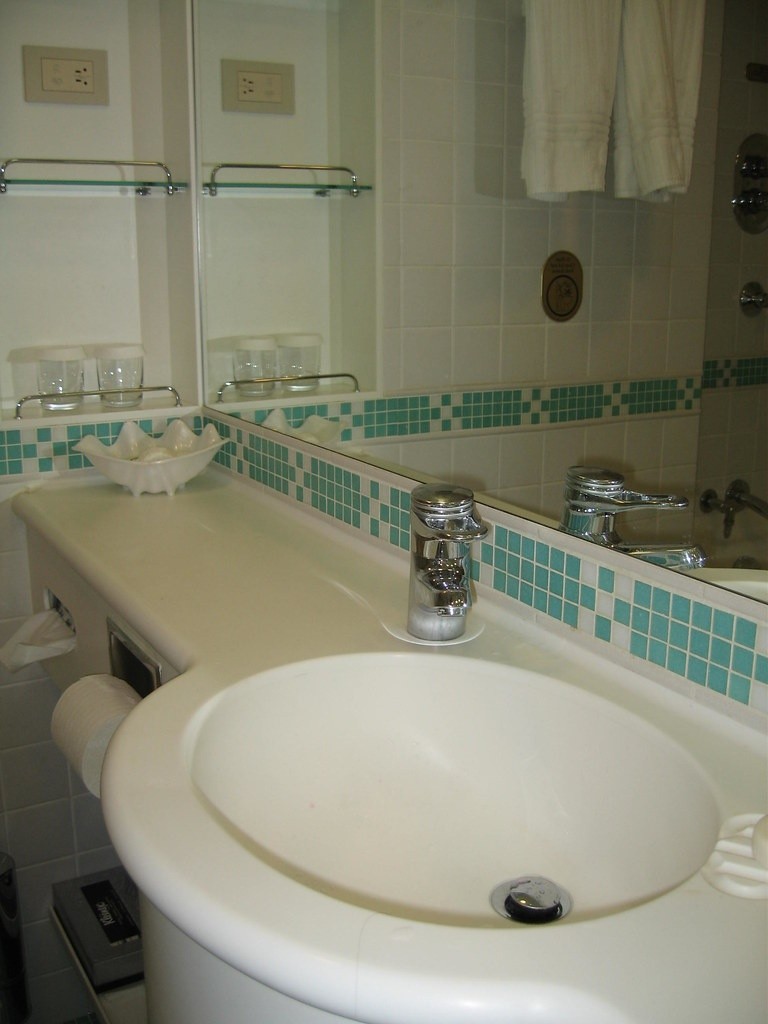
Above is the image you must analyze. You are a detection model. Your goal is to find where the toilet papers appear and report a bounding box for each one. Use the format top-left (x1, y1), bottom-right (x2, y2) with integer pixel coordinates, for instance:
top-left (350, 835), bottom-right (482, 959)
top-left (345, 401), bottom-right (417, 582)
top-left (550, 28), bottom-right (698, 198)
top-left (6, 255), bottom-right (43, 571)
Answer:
top-left (50, 673), bottom-right (143, 799)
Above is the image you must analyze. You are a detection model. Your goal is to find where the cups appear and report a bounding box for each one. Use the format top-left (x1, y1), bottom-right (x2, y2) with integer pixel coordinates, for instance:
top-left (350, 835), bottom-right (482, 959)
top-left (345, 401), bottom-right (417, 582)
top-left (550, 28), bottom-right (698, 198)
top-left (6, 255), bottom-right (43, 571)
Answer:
top-left (37, 347), bottom-right (83, 410)
top-left (236, 339), bottom-right (274, 393)
top-left (279, 337), bottom-right (321, 390)
top-left (97, 346), bottom-right (145, 407)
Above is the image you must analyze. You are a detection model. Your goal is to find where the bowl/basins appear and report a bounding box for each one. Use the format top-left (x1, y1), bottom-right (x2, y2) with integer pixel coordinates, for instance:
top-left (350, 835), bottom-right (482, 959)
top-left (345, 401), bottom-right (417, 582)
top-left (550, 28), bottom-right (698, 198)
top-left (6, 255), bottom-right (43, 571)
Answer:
top-left (72, 421), bottom-right (230, 496)
top-left (262, 409), bottom-right (344, 446)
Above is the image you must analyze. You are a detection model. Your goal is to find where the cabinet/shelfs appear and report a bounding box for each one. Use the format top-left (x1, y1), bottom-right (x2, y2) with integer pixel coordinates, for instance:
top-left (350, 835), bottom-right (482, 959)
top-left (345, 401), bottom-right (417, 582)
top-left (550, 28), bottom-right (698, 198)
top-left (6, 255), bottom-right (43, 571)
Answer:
top-left (0, 0), bottom-right (204, 431)
top-left (192, 0), bottom-right (384, 412)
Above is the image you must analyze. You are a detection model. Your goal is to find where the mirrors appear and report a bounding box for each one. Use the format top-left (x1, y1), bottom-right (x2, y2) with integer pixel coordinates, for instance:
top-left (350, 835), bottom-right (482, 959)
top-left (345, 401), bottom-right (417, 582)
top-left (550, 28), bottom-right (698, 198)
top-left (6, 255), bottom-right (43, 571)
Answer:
top-left (203, 0), bottom-right (768, 607)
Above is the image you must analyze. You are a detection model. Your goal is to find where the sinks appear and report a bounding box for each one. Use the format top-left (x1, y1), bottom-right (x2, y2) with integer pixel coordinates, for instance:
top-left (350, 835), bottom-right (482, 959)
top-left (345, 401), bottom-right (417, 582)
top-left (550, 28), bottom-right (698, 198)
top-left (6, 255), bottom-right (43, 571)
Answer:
top-left (684, 568), bottom-right (768, 604)
top-left (186, 649), bottom-right (724, 931)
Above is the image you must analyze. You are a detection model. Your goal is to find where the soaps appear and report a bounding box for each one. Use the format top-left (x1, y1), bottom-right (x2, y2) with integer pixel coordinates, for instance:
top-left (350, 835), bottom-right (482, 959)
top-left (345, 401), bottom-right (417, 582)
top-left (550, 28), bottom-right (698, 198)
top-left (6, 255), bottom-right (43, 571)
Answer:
top-left (751, 813), bottom-right (768, 875)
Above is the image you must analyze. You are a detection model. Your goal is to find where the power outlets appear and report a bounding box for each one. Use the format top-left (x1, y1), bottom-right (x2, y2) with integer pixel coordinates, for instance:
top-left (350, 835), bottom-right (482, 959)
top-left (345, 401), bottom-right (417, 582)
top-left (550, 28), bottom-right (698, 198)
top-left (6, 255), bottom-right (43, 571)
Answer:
top-left (221, 59), bottom-right (296, 116)
top-left (22, 44), bottom-right (110, 106)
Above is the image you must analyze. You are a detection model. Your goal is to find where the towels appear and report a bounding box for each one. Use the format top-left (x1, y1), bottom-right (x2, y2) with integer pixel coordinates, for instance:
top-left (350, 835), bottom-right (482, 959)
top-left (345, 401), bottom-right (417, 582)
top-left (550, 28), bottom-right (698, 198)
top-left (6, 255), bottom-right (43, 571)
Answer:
top-left (520, 0), bottom-right (707, 203)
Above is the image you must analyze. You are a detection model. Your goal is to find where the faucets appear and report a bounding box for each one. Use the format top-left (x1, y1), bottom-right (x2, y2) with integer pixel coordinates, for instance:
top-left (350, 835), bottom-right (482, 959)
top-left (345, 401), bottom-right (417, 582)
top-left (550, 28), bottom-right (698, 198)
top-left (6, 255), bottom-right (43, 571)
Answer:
top-left (407, 483), bottom-right (491, 641)
top-left (561, 465), bottom-right (708, 573)
